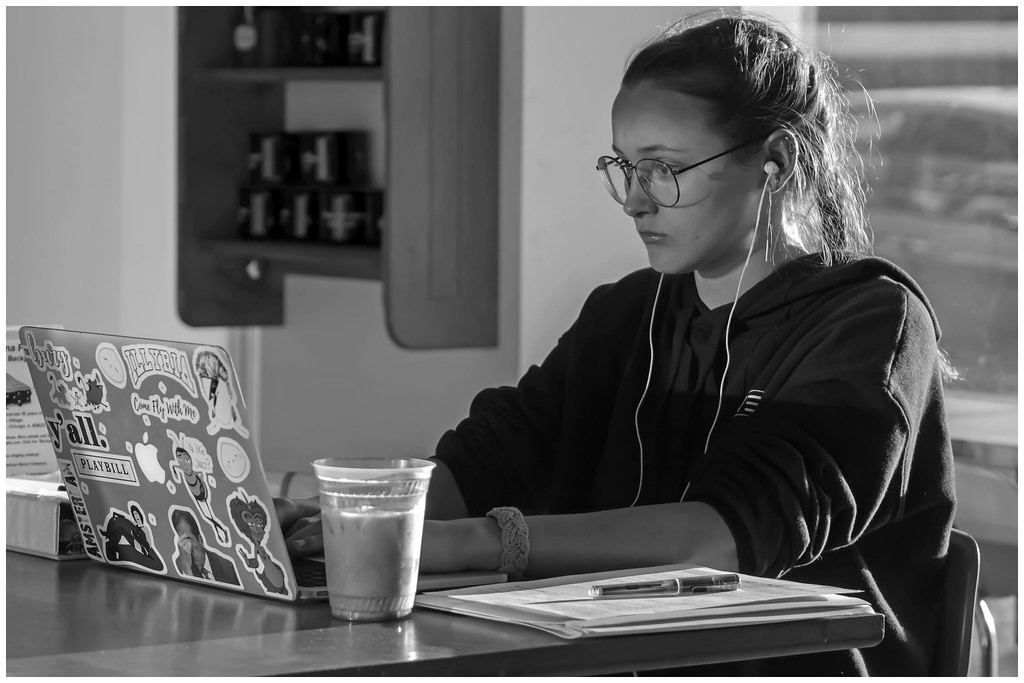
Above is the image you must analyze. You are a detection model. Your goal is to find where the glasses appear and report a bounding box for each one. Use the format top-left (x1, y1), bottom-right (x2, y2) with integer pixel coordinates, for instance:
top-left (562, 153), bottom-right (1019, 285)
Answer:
top-left (596, 130), bottom-right (777, 207)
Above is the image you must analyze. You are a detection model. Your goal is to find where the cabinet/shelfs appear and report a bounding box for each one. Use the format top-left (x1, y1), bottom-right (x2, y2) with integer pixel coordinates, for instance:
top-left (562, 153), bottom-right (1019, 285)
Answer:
top-left (174, 6), bottom-right (498, 355)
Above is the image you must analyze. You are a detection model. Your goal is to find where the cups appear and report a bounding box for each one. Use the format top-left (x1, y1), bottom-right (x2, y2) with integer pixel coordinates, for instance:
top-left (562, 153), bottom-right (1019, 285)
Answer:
top-left (313, 456), bottom-right (437, 622)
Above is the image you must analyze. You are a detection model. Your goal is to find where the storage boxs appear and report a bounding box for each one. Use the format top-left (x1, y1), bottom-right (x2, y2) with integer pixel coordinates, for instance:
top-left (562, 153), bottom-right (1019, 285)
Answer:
top-left (6, 479), bottom-right (91, 561)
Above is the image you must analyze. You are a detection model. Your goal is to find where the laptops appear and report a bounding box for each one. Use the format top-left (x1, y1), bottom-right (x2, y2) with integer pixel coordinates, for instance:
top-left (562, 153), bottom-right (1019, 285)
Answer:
top-left (20, 326), bottom-right (508, 601)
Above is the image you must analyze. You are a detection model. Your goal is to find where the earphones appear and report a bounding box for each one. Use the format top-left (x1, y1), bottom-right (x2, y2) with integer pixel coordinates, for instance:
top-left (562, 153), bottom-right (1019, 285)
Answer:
top-left (764, 162), bottom-right (778, 174)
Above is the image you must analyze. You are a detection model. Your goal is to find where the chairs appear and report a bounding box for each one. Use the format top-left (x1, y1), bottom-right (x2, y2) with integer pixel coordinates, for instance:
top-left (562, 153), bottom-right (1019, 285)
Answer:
top-left (938, 528), bottom-right (998, 677)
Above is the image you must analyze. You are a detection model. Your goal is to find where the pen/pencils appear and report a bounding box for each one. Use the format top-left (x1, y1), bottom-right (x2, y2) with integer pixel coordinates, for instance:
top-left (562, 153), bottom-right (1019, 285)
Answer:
top-left (590, 574), bottom-right (740, 599)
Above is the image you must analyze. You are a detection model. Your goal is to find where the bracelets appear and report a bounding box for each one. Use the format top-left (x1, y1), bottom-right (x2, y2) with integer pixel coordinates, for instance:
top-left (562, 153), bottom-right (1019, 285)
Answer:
top-left (488, 506), bottom-right (530, 580)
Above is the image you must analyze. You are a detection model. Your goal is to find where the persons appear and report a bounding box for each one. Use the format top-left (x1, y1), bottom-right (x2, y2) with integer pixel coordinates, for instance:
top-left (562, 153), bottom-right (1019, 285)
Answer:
top-left (165, 506), bottom-right (241, 587)
top-left (274, 16), bottom-right (958, 676)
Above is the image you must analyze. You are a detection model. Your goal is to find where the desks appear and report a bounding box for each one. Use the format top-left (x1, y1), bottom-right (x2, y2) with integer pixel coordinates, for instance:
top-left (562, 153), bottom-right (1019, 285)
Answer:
top-left (6, 554), bottom-right (886, 677)
